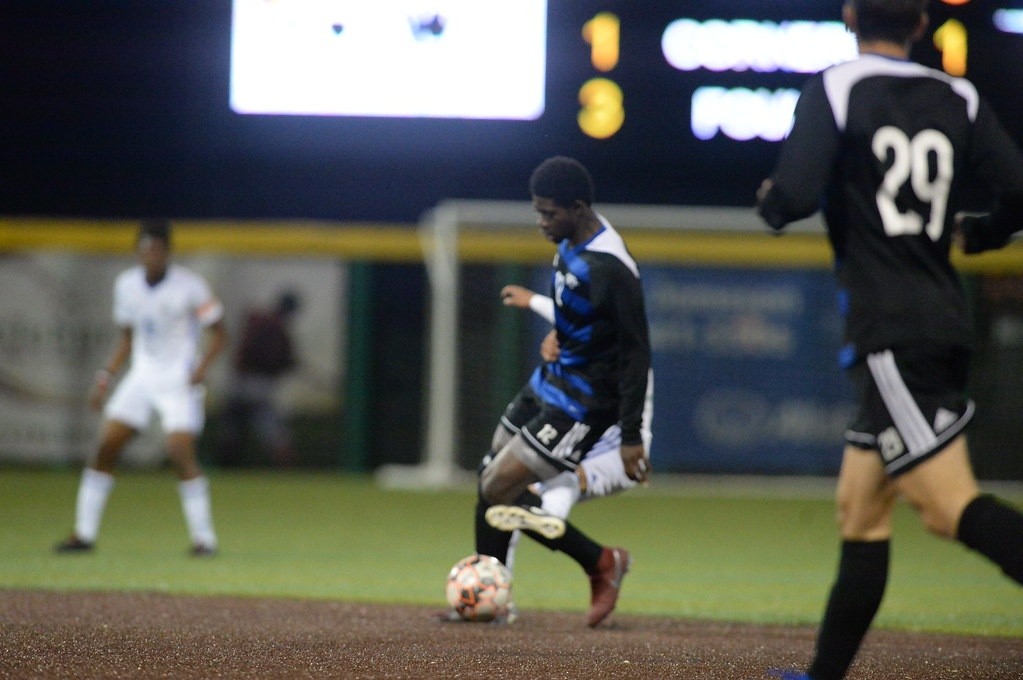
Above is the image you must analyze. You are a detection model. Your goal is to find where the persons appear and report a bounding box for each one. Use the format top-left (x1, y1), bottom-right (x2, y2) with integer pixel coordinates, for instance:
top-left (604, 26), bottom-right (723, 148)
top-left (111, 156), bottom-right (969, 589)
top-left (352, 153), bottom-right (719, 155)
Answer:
top-left (53, 209), bottom-right (226, 555)
top-left (229, 287), bottom-right (304, 462)
top-left (447, 157), bottom-right (657, 628)
top-left (752, 1), bottom-right (1023, 680)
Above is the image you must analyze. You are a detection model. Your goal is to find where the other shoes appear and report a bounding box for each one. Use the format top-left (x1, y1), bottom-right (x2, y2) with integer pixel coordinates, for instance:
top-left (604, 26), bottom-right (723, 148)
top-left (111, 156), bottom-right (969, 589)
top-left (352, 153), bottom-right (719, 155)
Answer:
top-left (55, 536), bottom-right (92, 552)
top-left (487, 504), bottom-right (566, 537)
top-left (193, 546), bottom-right (215, 559)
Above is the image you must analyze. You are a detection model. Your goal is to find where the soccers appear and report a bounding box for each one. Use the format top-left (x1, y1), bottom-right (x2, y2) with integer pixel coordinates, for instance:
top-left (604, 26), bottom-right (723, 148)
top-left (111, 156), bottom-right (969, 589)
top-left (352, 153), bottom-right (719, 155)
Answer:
top-left (448, 554), bottom-right (514, 621)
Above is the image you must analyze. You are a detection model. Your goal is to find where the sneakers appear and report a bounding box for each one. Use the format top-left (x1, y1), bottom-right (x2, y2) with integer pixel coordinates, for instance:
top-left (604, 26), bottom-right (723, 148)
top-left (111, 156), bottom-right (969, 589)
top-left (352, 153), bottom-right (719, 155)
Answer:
top-left (585, 546), bottom-right (631, 629)
top-left (432, 603), bottom-right (516, 625)
